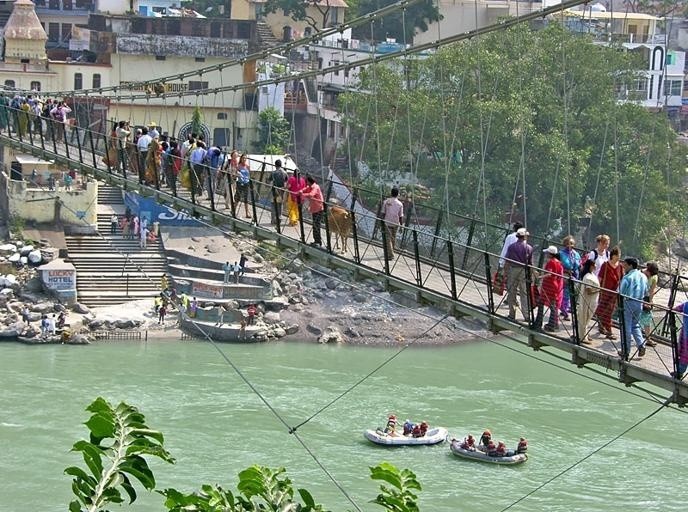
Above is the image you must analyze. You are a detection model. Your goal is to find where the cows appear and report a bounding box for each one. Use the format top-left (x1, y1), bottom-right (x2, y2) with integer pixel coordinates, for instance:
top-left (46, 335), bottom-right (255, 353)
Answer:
top-left (328, 205), bottom-right (356, 254)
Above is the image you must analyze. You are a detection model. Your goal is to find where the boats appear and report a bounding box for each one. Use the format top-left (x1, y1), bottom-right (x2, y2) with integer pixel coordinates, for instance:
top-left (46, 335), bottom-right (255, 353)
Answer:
top-left (448, 436), bottom-right (530, 466)
top-left (363, 423), bottom-right (450, 449)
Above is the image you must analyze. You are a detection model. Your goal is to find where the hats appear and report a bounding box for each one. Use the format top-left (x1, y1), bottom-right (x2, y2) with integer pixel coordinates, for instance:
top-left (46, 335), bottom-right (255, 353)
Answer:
top-left (515, 227), bottom-right (530, 237)
top-left (147, 122), bottom-right (156, 127)
top-left (542, 245), bottom-right (558, 256)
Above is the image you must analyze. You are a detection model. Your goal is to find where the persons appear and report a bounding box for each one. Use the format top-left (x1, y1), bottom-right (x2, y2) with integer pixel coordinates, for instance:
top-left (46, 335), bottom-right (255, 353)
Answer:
top-left (302, 178), bottom-right (324, 247)
top-left (222, 261), bottom-right (232, 283)
top-left (286, 169), bottom-right (304, 226)
top-left (265, 160), bottom-right (286, 224)
top-left (150, 273), bottom-right (261, 342)
top-left (492, 221), bottom-right (688, 387)
top-left (239, 253), bottom-right (248, 274)
top-left (377, 415), bottom-right (530, 458)
top-left (20, 303), bottom-right (72, 346)
top-left (99, 116), bottom-right (258, 247)
top-left (0, 93), bottom-right (73, 145)
top-left (381, 187), bottom-right (405, 260)
top-left (27, 166), bottom-right (80, 194)
top-left (231, 260), bottom-right (242, 282)
